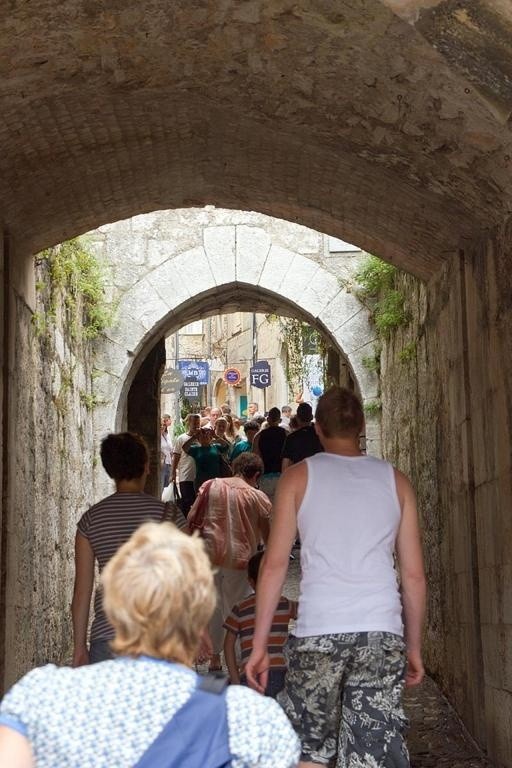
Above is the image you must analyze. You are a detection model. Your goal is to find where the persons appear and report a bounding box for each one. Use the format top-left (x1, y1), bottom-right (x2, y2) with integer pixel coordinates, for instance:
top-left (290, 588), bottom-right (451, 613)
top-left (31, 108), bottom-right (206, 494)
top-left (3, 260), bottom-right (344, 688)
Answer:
top-left (70, 431), bottom-right (215, 669)
top-left (186, 450), bottom-right (272, 677)
top-left (154, 401), bottom-right (332, 550)
top-left (220, 551), bottom-right (299, 700)
top-left (1, 519), bottom-right (303, 768)
top-left (244, 385), bottom-right (426, 767)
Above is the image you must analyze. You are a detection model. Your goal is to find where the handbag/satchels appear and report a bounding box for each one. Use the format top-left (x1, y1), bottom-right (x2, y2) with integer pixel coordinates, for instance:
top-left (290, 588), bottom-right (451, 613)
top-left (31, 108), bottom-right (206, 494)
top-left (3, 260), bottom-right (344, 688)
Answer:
top-left (190, 496), bottom-right (209, 537)
